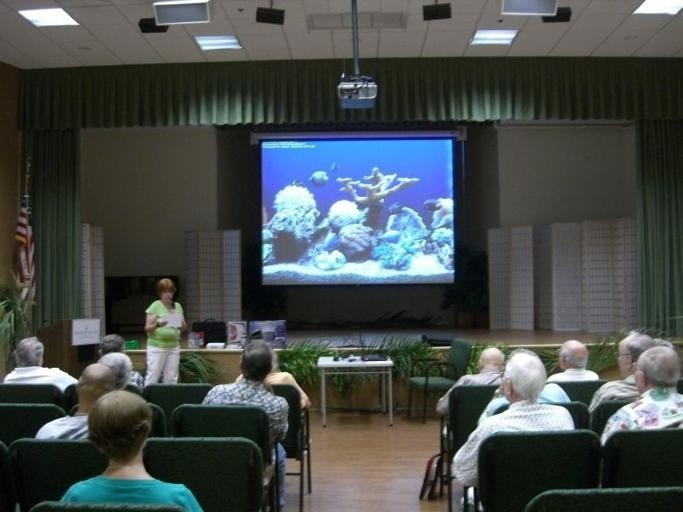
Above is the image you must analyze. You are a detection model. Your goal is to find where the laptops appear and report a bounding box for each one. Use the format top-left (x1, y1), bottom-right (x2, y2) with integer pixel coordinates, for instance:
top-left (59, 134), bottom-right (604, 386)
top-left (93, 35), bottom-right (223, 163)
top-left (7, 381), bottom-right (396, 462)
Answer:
top-left (360, 334), bottom-right (387, 361)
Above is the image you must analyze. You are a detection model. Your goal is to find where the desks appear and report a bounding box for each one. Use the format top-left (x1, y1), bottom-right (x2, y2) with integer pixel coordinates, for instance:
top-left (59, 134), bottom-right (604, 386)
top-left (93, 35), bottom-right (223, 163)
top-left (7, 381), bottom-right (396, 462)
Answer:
top-left (317, 356), bottom-right (395, 427)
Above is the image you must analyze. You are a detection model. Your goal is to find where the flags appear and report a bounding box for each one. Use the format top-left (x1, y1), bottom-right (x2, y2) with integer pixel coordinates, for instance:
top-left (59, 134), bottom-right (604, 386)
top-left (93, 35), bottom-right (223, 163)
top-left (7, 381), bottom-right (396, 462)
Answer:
top-left (11, 171), bottom-right (39, 306)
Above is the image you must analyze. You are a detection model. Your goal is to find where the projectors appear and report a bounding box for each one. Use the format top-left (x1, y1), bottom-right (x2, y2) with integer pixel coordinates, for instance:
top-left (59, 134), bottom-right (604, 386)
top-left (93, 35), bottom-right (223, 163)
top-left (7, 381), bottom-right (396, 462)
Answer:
top-left (336, 75), bottom-right (378, 109)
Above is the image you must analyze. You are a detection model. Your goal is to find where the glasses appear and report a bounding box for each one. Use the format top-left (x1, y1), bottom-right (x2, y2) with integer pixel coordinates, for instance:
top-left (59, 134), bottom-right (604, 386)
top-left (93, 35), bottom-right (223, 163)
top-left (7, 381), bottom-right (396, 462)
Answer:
top-left (613, 351), bottom-right (632, 360)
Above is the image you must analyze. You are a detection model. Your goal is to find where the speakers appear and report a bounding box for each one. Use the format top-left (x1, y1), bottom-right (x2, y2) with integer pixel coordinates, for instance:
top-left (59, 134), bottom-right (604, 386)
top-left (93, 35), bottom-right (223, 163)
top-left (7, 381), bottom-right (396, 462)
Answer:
top-left (501, 0), bottom-right (558, 16)
top-left (153, 0), bottom-right (213, 26)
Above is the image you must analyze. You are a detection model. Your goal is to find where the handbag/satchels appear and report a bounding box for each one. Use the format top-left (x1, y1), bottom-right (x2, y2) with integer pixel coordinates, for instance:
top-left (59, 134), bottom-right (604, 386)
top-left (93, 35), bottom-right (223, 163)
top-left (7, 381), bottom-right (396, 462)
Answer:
top-left (192, 318), bottom-right (227, 348)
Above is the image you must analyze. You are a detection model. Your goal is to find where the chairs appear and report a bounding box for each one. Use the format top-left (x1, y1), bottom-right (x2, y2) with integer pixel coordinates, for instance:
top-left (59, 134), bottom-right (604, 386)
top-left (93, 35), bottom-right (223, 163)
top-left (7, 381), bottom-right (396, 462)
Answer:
top-left (0, 383), bottom-right (311, 511)
top-left (408, 338), bottom-right (473, 424)
top-left (440, 379), bottom-right (683, 512)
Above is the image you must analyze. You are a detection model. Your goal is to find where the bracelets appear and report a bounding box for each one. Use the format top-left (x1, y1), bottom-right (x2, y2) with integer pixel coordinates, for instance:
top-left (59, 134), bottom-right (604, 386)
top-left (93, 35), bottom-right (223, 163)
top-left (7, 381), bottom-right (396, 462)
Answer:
top-left (154, 320), bottom-right (160, 327)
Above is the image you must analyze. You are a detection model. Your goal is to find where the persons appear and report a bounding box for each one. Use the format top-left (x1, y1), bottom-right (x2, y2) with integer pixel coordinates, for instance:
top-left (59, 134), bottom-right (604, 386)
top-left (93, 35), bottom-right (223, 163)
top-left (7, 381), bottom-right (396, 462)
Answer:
top-left (545, 340), bottom-right (600, 382)
top-left (433, 346), bottom-right (506, 452)
top-left (478, 348), bottom-right (571, 426)
top-left (99, 334), bottom-right (144, 386)
top-left (97, 352), bottom-right (133, 388)
top-left (1, 337), bottom-right (79, 392)
top-left (236, 346), bottom-right (310, 408)
top-left (60, 389), bottom-right (204, 511)
top-left (32, 363), bottom-right (115, 440)
top-left (589, 331), bottom-right (683, 443)
top-left (450, 349), bottom-right (575, 511)
top-left (143, 278), bottom-right (189, 384)
top-left (202, 339), bottom-right (289, 510)
